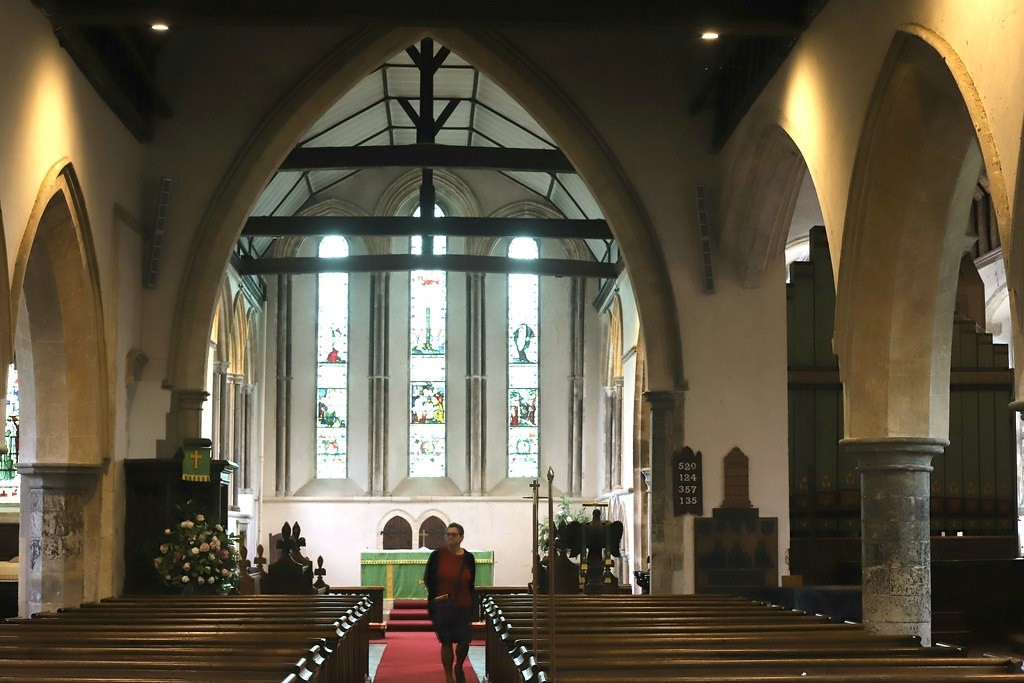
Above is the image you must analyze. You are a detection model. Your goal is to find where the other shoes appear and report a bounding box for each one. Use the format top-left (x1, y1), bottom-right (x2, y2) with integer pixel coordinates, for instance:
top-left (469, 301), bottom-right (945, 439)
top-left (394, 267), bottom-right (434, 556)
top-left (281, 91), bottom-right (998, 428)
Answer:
top-left (454, 664), bottom-right (465, 683)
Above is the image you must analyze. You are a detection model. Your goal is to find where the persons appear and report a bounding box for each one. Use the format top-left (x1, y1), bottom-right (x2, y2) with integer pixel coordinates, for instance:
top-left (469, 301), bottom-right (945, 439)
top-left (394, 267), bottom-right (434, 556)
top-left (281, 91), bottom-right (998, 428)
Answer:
top-left (424, 523), bottom-right (475, 683)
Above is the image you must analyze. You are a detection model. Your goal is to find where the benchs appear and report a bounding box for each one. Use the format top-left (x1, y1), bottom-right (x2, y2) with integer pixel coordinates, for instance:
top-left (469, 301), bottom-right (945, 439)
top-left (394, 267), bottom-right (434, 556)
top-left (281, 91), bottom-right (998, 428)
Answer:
top-left (484, 592), bottom-right (1024, 683)
top-left (0, 589), bottom-right (374, 683)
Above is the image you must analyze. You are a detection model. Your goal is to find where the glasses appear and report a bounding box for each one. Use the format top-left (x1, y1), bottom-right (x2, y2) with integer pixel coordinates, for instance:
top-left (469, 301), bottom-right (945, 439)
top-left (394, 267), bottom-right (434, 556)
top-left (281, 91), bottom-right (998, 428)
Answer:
top-left (446, 532), bottom-right (459, 537)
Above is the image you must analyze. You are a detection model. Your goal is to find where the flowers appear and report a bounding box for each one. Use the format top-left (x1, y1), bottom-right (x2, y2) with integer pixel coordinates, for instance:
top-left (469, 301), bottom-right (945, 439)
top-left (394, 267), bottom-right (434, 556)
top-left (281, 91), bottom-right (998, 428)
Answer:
top-left (539, 497), bottom-right (591, 554)
top-left (155, 515), bottom-right (239, 589)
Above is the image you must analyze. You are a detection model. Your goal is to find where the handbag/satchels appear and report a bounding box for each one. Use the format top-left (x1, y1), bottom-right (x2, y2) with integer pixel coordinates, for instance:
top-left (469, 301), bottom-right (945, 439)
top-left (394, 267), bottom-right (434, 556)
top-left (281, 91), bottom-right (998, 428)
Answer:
top-left (436, 601), bottom-right (459, 622)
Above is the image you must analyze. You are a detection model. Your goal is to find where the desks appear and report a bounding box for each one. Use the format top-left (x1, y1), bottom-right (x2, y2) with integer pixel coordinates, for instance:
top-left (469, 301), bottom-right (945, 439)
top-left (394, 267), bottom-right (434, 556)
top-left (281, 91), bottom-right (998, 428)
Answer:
top-left (359, 551), bottom-right (494, 600)
